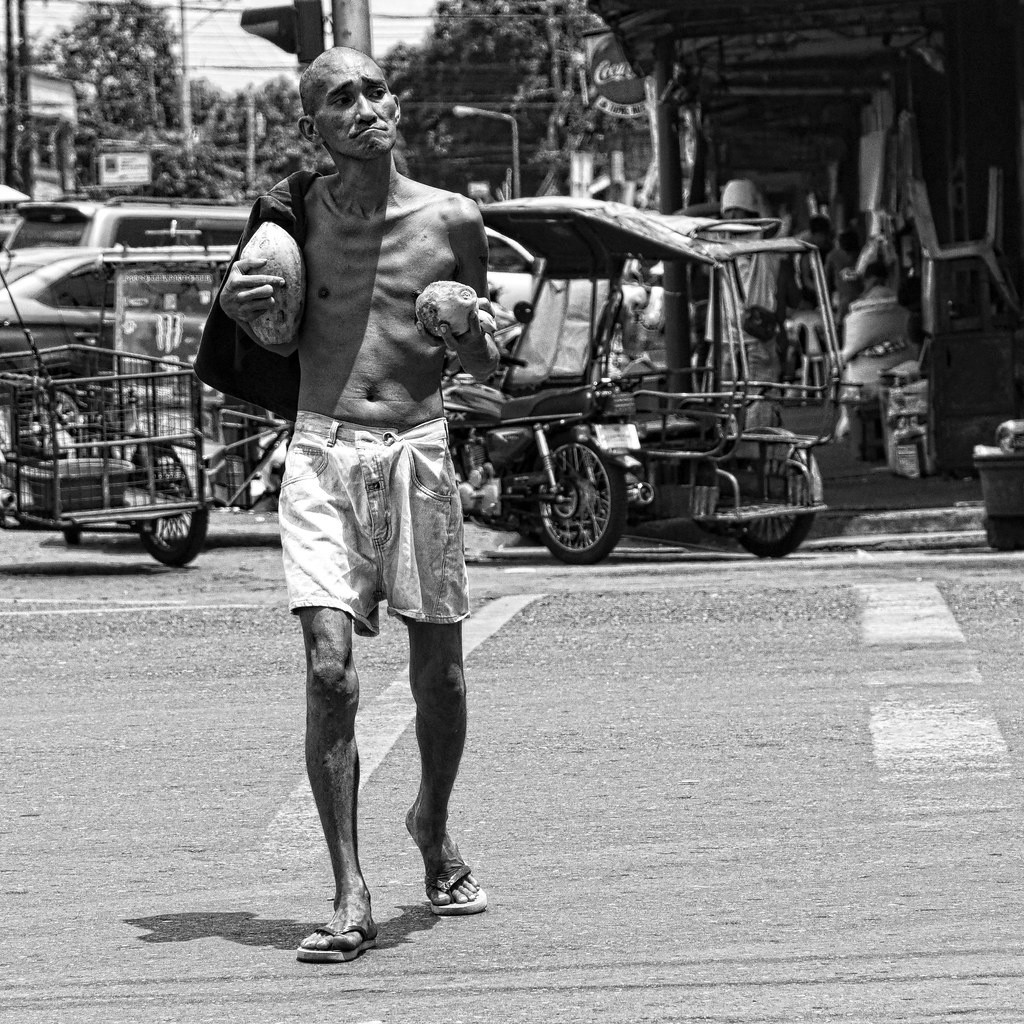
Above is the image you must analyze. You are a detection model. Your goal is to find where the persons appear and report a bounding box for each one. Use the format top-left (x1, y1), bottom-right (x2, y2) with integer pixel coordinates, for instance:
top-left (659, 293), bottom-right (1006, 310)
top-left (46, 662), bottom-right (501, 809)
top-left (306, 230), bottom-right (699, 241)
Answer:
top-left (218, 46), bottom-right (503, 963)
top-left (702, 176), bottom-right (903, 394)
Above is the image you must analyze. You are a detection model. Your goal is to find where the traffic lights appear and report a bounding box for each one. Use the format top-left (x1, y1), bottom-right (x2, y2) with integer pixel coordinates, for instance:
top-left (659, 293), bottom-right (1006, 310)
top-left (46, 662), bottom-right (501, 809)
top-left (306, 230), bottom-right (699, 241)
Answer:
top-left (238, 0), bottom-right (322, 66)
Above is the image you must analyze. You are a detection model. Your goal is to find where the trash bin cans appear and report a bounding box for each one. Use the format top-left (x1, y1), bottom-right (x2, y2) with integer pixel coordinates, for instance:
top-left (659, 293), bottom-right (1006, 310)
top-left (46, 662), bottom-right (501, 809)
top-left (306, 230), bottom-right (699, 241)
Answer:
top-left (972, 443), bottom-right (1024, 551)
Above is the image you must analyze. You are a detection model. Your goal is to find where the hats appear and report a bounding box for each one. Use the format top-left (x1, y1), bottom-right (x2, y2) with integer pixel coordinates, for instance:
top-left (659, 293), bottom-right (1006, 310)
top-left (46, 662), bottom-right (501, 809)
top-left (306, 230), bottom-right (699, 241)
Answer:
top-left (721, 180), bottom-right (759, 215)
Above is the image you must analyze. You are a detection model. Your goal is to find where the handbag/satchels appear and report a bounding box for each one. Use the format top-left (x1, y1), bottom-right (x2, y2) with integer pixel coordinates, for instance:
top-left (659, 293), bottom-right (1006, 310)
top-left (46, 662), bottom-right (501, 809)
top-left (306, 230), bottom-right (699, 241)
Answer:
top-left (741, 302), bottom-right (782, 340)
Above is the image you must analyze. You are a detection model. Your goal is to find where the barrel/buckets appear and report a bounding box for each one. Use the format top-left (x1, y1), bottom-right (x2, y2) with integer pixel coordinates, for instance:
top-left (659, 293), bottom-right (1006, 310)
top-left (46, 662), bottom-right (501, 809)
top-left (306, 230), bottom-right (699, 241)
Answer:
top-left (973, 454), bottom-right (1024, 550)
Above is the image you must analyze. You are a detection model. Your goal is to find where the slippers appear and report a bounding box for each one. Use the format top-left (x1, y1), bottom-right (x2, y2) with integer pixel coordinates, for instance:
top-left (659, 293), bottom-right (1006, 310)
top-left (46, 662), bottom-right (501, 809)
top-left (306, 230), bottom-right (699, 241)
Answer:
top-left (425, 865), bottom-right (488, 916)
top-left (296, 926), bottom-right (376, 961)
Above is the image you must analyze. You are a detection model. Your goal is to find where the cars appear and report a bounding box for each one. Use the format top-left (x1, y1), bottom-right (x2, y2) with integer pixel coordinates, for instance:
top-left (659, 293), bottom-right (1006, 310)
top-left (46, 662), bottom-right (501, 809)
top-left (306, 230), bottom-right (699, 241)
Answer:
top-left (2, 185), bottom-right (867, 567)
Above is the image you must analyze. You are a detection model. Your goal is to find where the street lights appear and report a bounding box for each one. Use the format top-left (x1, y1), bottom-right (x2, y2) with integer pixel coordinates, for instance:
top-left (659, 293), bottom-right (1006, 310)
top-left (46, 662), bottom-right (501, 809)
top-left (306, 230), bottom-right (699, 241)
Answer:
top-left (451, 104), bottom-right (520, 199)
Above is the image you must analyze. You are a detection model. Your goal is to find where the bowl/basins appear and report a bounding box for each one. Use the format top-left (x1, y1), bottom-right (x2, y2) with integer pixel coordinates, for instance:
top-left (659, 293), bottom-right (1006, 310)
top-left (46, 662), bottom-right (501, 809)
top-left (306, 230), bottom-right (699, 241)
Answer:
top-left (21, 458), bottom-right (133, 511)
